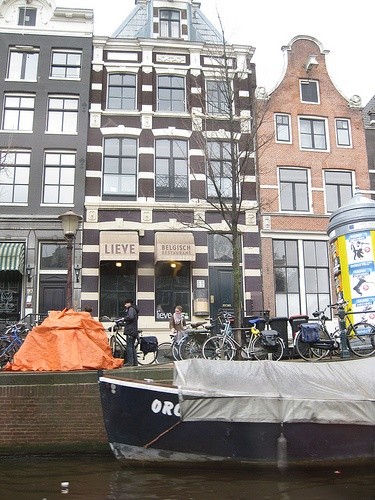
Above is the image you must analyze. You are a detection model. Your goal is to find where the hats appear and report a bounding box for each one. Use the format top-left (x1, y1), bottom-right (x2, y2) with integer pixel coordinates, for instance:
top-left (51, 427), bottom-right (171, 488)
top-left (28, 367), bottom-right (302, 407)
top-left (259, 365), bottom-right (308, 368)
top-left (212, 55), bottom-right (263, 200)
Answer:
top-left (124, 298), bottom-right (133, 303)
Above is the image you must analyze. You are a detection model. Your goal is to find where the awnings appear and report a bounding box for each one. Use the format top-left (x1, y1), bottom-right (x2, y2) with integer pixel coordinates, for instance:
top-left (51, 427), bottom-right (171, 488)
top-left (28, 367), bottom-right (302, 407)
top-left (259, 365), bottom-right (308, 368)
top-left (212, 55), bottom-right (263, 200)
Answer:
top-left (0, 243), bottom-right (24, 276)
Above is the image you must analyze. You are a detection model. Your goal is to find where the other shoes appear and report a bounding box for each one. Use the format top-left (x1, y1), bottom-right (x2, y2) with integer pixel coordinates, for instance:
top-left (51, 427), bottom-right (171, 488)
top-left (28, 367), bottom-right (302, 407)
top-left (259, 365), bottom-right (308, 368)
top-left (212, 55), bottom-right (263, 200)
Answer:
top-left (125, 361), bottom-right (138, 366)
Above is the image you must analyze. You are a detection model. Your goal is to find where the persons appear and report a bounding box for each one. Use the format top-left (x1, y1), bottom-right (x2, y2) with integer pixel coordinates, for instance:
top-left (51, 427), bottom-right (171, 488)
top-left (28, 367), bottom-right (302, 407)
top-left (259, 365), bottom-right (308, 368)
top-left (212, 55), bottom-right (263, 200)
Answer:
top-left (169, 305), bottom-right (186, 361)
top-left (122, 299), bottom-right (139, 366)
top-left (335, 286), bottom-right (343, 303)
top-left (332, 252), bottom-right (340, 267)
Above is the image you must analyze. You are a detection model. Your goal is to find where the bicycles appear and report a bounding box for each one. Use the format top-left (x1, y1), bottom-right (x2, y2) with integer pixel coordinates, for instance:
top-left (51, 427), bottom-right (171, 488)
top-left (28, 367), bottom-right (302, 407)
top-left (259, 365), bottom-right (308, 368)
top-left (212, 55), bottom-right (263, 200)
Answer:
top-left (153, 308), bottom-right (234, 364)
top-left (109, 319), bottom-right (159, 365)
top-left (0, 320), bottom-right (42, 366)
top-left (201, 310), bottom-right (286, 361)
top-left (293, 301), bottom-right (375, 362)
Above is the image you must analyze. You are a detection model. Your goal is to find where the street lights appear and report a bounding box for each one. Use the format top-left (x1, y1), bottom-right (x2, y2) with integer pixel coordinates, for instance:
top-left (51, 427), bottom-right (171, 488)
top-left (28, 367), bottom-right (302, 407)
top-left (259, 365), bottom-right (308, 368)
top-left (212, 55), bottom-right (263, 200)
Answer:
top-left (57, 208), bottom-right (84, 311)
